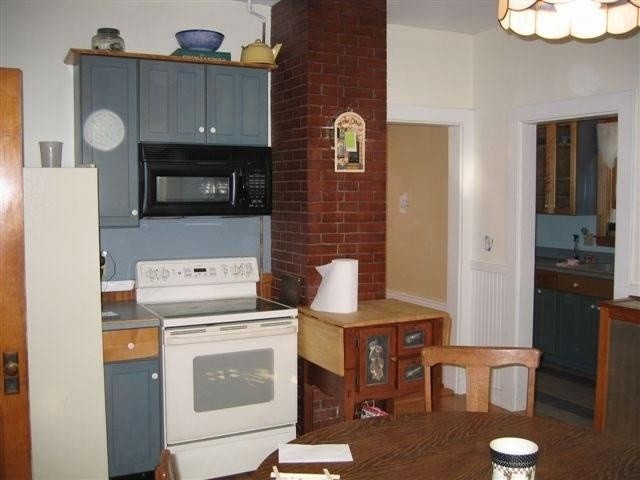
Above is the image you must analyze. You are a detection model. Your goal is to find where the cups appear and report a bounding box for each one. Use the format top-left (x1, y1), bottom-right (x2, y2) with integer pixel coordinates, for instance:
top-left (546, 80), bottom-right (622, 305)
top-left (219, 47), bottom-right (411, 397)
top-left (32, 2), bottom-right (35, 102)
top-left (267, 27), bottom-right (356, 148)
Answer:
top-left (38, 140), bottom-right (63, 168)
top-left (489, 435), bottom-right (541, 480)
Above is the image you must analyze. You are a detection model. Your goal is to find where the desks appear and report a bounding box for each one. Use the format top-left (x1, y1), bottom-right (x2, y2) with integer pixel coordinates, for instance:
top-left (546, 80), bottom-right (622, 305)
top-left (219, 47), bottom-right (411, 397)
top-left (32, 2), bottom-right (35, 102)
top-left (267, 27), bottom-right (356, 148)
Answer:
top-left (298, 298), bottom-right (451, 436)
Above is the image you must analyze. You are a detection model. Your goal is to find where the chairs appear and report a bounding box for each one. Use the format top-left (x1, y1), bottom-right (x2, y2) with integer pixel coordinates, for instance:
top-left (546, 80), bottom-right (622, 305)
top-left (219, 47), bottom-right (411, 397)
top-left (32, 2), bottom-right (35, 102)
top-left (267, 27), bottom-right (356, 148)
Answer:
top-left (420, 345), bottom-right (544, 414)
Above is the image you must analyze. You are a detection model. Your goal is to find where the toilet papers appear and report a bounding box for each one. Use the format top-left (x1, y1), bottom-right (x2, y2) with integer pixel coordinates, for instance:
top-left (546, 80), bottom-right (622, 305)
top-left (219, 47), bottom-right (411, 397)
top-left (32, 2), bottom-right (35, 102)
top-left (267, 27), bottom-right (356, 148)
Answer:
top-left (310, 259), bottom-right (358, 314)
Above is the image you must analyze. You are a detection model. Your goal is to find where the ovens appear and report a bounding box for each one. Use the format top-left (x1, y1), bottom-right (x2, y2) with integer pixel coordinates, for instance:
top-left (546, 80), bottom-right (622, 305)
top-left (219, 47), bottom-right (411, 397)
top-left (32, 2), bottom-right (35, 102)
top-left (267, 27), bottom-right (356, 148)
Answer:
top-left (159, 313), bottom-right (301, 479)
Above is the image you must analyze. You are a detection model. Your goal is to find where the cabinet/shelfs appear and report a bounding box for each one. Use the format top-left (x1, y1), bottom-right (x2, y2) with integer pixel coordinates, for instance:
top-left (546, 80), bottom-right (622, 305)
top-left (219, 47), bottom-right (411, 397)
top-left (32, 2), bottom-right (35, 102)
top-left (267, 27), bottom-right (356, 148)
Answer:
top-left (64, 48), bottom-right (272, 228)
top-left (102, 318), bottom-right (162, 480)
top-left (533, 264), bottom-right (613, 388)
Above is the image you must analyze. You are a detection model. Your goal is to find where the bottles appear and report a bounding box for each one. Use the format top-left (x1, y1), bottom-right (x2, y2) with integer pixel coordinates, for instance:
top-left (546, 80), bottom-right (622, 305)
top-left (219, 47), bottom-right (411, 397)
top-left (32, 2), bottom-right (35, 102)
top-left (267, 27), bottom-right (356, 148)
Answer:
top-left (91, 28), bottom-right (126, 54)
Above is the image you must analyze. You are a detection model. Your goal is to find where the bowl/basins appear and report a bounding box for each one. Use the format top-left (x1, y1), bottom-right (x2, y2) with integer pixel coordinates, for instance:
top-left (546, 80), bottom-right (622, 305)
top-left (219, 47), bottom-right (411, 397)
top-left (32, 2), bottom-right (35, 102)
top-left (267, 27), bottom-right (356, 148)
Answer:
top-left (174, 29), bottom-right (225, 52)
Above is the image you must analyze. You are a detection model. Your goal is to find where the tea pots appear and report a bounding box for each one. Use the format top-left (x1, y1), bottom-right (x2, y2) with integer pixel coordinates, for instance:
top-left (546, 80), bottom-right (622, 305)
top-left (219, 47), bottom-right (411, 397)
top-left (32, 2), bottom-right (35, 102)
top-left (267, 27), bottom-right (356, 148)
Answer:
top-left (238, 38), bottom-right (285, 65)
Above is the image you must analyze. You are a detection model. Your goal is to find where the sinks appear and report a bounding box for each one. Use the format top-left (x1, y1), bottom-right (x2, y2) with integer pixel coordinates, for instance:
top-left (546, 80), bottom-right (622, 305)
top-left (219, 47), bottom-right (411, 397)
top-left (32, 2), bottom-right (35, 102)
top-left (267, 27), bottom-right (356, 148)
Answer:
top-left (563, 261), bottom-right (614, 275)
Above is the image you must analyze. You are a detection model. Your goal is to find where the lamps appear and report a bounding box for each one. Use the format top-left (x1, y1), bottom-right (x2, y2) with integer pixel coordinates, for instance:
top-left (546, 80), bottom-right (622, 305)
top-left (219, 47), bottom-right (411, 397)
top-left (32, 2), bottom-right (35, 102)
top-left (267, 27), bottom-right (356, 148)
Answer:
top-left (496, 0), bottom-right (640, 46)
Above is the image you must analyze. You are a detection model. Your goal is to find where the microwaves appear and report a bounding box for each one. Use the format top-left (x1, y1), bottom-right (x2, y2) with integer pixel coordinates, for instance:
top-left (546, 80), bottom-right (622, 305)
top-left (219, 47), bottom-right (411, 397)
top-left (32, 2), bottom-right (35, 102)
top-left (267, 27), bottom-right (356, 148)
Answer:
top-left (136, 141), bottom-right (274, 222)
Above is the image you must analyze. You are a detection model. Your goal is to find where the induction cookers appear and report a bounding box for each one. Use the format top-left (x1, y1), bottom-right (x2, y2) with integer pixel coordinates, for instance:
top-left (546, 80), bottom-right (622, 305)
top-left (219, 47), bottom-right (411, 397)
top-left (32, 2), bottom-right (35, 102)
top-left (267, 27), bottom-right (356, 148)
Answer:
top-left (140, 294), bottom-right (299, 327)
